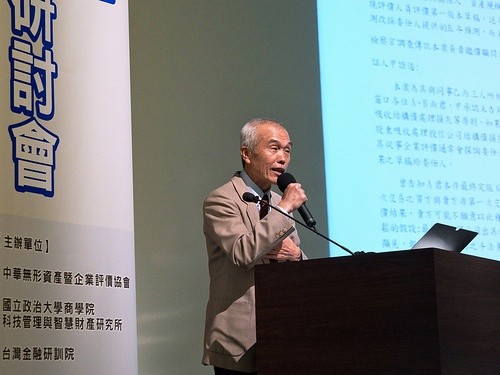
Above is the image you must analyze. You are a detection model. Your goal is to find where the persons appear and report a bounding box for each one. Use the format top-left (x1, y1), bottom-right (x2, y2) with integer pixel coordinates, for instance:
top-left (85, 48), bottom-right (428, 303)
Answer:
top-left (201, 119), bottom-right (309, 375)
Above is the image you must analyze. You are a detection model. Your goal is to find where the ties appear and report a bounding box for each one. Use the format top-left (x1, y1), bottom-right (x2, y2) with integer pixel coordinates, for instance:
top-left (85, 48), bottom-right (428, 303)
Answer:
top-left (259, 194), bottom-right (269, 220)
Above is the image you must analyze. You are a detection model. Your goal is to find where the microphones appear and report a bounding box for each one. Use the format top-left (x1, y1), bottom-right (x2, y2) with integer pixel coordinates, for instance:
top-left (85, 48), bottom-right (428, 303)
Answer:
top-left (277, 172), bottom-right (317, 231)
top-left (242, 192), bottom-right (356, 255)
